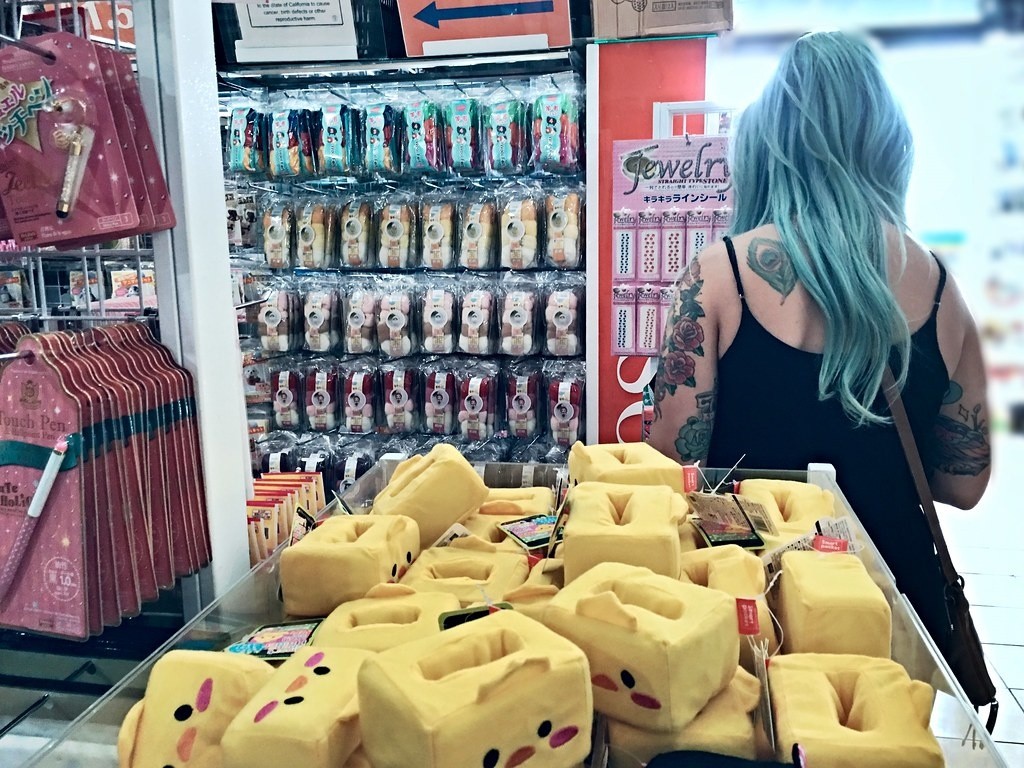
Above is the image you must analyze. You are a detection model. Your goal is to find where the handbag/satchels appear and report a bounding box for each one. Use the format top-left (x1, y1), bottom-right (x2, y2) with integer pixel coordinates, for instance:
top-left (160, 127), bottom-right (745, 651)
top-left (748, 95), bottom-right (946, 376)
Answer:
top-left (945, 574), bottom-right (996, 708)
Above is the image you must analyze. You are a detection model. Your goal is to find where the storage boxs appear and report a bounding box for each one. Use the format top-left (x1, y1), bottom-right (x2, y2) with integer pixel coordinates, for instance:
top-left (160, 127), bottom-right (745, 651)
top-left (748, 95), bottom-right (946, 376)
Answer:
top-left (22, 449), bottom-right (1015, 768)
top-left (212, 0), bottom-right (732, 64)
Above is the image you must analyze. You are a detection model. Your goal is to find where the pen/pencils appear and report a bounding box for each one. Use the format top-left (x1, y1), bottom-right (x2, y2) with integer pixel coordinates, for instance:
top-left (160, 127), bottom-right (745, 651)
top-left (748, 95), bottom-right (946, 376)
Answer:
top-left (0, 439), bottom-right (69, 605)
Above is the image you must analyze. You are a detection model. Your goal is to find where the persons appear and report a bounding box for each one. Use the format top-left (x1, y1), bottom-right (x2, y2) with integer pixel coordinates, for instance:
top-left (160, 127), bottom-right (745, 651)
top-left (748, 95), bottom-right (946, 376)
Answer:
top-left (643, 28), bottom-right (993, 708)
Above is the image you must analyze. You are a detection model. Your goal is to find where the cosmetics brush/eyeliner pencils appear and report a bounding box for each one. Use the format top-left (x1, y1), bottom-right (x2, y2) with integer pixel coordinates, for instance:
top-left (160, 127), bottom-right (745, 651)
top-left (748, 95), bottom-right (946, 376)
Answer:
top-left (0, 435), bottom-right (69, 607)
